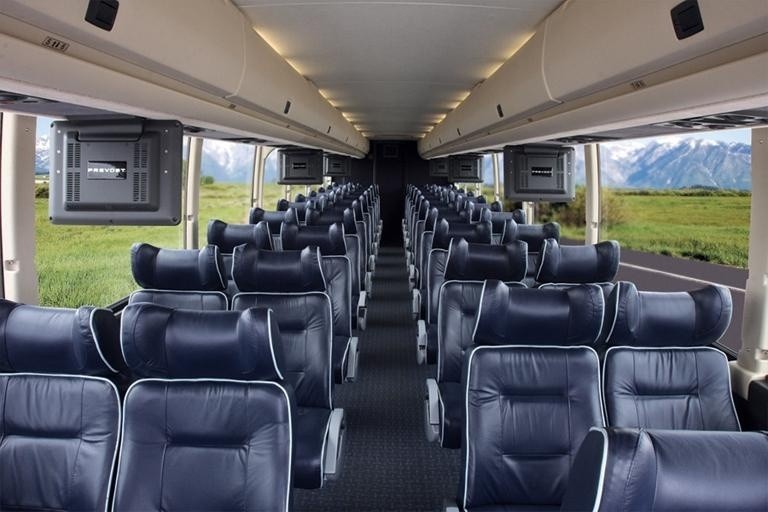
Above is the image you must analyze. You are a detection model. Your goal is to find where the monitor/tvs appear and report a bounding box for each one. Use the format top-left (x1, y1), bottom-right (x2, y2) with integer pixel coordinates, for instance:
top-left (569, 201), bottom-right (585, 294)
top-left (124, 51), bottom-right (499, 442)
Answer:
top-left (430, 159), bottom-right (449, 177)
top-left (323, 154), bottom-right (352, 177)
top-left (277, 148), bottom-right (323, 184)
top-left (503, 145), bottom-right (575, 202)
top-left (47, 116), bottom-right (183, 225)
top-left (448, 156), bottom-right (484, 182)
